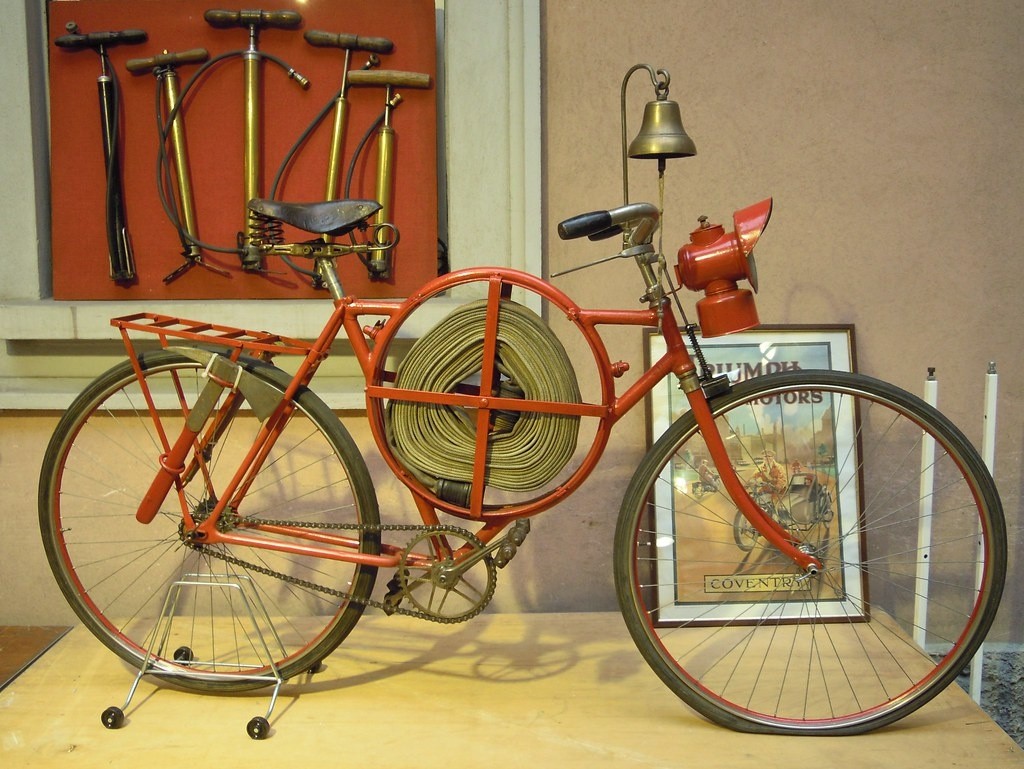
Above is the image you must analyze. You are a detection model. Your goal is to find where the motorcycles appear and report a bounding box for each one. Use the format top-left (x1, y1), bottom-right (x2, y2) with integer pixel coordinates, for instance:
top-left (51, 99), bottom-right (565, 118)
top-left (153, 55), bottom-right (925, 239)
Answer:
top-left (691, 472), bottom-right (721, 498)
top-left (732, 470), bottom-right (836, 552)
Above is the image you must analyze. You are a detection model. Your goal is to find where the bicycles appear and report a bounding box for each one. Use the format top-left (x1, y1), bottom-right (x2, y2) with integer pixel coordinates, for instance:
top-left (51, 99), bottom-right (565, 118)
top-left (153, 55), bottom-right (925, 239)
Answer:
top-left (29, 194), bottom-right (1012, 738)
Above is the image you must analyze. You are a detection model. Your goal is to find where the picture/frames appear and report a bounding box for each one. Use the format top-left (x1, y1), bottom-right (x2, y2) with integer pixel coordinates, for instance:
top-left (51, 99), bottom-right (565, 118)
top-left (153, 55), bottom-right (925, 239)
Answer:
top-left (640, 322), bottom-right (872, 629)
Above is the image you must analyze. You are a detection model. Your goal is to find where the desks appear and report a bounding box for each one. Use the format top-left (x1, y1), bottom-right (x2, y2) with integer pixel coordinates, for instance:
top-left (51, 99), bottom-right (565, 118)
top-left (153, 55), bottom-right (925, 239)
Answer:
top-left (1, 605), bottom-right (1024, 769)
